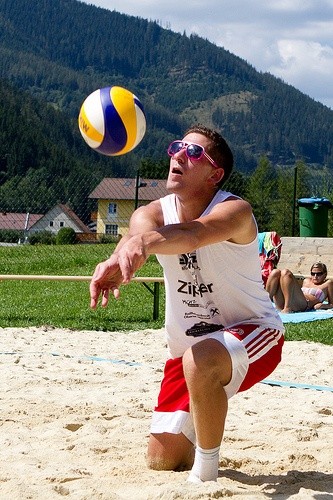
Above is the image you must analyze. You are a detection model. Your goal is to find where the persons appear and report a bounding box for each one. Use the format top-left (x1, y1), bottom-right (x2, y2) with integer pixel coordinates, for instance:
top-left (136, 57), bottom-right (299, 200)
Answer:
top-left (89, 124), bottom-right (285, 484)
top-left (264, 263), bottom-right (333, 313)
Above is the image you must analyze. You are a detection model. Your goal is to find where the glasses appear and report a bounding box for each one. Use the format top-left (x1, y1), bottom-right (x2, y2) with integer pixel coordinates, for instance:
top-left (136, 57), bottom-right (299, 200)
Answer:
top-left (167, 140), bottom-right (218, 168)
top-left (311, 272), bottom-right (324, 276)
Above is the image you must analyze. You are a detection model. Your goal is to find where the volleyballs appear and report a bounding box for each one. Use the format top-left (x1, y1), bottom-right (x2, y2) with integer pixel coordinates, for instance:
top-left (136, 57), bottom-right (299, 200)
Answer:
top-left (77, 85), bottom-right (147, 159)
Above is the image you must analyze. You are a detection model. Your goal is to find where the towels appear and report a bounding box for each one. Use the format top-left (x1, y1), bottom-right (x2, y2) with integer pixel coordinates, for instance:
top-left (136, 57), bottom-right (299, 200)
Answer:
top-left (258, 231), bottom-right (282, 286)
